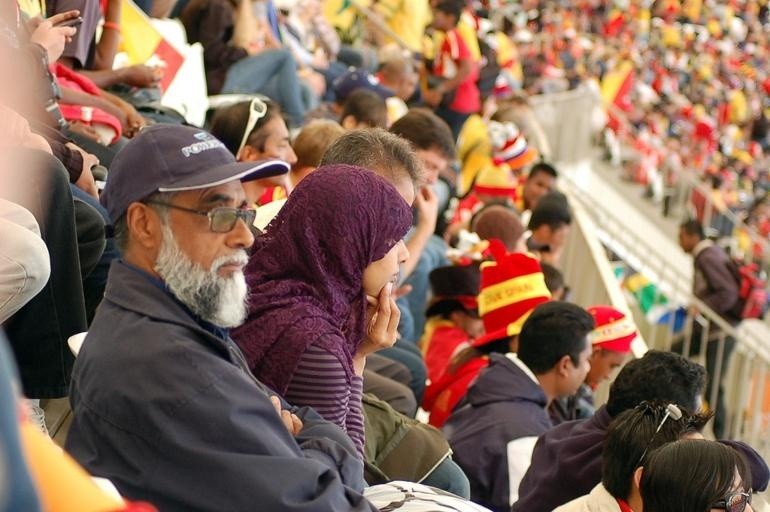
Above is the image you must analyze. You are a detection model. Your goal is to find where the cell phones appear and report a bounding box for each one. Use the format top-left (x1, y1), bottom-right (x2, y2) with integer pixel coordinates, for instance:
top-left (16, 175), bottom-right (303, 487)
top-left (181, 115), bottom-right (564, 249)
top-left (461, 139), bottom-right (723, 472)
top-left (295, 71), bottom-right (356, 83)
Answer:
top-left (53, 16), bottom-right (84, 27)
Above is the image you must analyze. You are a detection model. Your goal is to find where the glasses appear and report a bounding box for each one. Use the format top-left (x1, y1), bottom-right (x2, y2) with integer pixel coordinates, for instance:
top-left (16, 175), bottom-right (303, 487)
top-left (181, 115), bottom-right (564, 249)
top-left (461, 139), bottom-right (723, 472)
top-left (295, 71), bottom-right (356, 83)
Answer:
top-left (235, 96), bottom-right (268, 162)
top-left (708, 486), bottom-right (754, 511)
top-left (631, 402), bottom-right (684, 474)
top-left (139, 199), bottom-right (258, 234)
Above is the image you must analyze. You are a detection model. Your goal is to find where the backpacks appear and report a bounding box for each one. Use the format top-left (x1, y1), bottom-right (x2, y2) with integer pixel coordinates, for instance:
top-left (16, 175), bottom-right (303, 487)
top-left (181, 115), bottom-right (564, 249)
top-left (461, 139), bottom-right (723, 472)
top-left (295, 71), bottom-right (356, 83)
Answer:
top-left (725, 253), bottom-right (770, 323)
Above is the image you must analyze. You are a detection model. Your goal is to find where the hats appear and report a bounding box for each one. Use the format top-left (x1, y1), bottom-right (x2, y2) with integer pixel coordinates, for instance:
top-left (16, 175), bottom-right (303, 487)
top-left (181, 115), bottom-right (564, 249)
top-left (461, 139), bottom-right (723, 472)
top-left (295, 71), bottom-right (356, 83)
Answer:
top-left (490, 120), bottom-right (540, 171)
top-left (469, 252), bottom-right (556, 351)
top-left (423, 265), bottom-right (484, 320)
top-left (332, 68), bottom-right (396, 106)
top-left (581, 304), bottom-right (640, 354)
top-left (97, 121), bottom-right (292, 241)
top-left (472, 155), bottom-right (520, 197)
